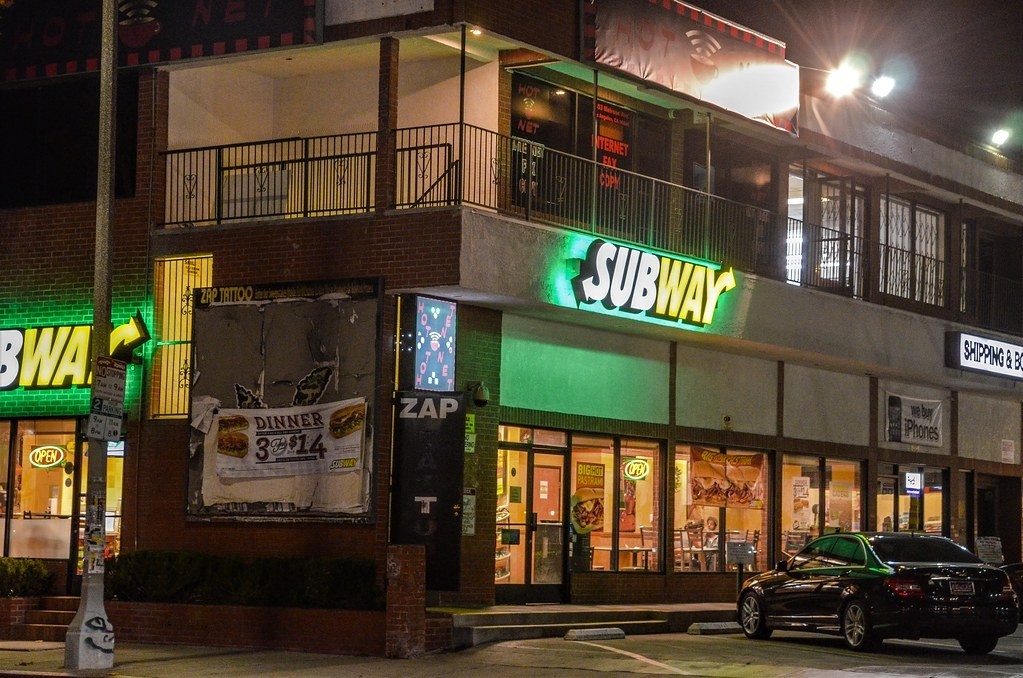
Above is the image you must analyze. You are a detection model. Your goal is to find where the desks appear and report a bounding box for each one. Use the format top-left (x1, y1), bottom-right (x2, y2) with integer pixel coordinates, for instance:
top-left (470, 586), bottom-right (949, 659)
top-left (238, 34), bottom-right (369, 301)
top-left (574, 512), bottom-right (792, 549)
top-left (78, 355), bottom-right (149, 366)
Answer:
top-left (594, 547), bottom-right (652, 571)
top-left (676, 546), bottom-right (718, 555)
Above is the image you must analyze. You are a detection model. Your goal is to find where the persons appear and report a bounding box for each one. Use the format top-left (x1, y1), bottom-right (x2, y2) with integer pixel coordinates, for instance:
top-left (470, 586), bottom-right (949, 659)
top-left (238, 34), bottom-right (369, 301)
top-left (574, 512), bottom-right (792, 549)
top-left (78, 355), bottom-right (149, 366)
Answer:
top-left (688, 516), bottom-right (718, 572)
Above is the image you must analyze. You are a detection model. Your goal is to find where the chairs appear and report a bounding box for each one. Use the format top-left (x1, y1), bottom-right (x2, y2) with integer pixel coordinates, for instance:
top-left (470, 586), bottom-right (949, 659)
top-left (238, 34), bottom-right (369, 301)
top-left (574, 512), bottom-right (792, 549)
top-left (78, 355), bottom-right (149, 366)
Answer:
top-left (752, 529), bottom-right (760, 572)
top-left (784, 530), bottom-right (808, 561)
top-left (687, 527), bottom-right (709, 572)
top-left (673, 529), bottom-right (691, 573)
top-left (639, 526), bottom-right (653, 570)
top-left (589, 547), bottom-right (605, 571)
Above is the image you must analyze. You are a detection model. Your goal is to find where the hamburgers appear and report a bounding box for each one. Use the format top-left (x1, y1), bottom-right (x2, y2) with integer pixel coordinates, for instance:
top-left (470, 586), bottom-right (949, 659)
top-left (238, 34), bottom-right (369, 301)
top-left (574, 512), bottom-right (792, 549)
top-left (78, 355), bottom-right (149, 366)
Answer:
top-left (329, 403), bottom-right (365, 439)
top-left (216, 415), bottom-right (250, 458)
top-left (570, 488), bottom-right (603, 535)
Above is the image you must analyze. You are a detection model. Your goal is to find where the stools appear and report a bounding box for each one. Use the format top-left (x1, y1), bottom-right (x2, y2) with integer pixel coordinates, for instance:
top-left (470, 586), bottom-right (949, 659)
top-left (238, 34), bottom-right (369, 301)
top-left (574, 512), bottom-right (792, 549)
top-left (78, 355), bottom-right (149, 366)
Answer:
top-left (622, 566), bottom-right (647, 570)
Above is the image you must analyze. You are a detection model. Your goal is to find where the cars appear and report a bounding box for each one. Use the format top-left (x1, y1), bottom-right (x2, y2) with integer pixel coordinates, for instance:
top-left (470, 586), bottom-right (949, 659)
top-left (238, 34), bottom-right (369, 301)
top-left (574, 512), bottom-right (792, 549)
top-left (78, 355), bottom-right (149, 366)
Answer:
top-left (736, 531), bottom-right (1018, 653)
top-left (999, 562), bottom-right (1023, 624)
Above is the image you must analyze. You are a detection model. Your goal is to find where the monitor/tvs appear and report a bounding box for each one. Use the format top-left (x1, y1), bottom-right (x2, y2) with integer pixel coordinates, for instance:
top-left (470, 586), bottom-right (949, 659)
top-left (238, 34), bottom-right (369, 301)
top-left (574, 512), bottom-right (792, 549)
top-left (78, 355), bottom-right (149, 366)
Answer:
top-left (107, 441), bottom-right (124, 457)
